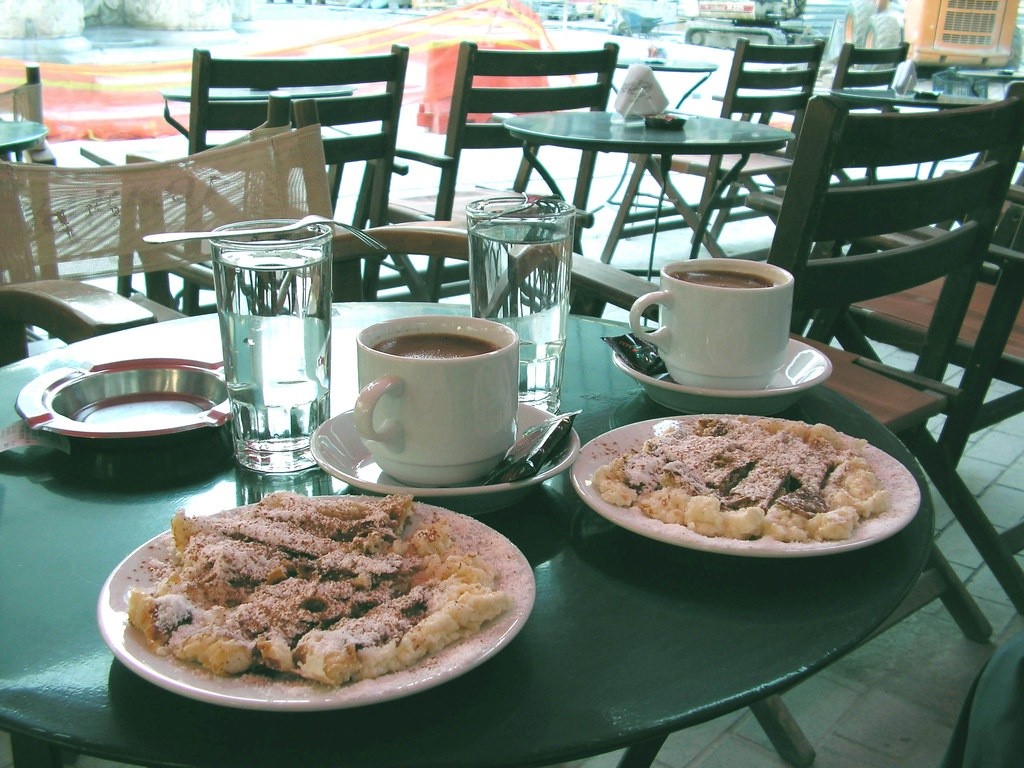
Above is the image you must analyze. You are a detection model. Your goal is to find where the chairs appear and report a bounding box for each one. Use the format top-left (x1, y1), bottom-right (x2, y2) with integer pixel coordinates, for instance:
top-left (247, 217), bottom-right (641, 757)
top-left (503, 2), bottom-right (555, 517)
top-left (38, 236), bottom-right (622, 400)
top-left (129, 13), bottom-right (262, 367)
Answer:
top-left (0, 42), bottom-right (1023, 768)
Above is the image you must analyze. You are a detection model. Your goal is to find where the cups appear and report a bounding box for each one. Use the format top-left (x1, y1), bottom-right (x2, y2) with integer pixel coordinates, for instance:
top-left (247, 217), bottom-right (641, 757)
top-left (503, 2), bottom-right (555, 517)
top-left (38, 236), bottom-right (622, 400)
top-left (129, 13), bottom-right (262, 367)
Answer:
top-left (208, 218), bottom-right (333, 472)
top-left (466, 197), bottom-right (577, 414)
top-left (351, 315), bottom-right (521, 489)
top-left (629, 258), bottom-right (795, 390)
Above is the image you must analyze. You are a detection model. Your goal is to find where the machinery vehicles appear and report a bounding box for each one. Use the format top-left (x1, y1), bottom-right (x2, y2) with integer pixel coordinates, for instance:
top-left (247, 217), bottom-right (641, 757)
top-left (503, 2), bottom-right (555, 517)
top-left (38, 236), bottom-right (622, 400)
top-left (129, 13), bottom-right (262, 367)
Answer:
top-left (678, 1), bottom-right (1024, 82)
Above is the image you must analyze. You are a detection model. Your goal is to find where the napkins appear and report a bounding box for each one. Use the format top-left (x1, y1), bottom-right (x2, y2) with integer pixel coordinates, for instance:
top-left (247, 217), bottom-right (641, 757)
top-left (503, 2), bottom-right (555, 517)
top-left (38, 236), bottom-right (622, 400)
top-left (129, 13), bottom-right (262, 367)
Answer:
top-left (614, 64), bottom-right (668, 118)
top-left (892, 59), bottom-right (917, 94)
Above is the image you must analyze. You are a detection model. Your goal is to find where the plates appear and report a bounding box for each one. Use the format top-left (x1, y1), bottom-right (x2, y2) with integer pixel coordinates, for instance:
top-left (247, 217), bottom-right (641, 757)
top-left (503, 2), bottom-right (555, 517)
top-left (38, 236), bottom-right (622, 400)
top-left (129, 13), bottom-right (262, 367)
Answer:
top-left (613, 327), bottom-right (834, 417)
top-left (571, 413), bottom-right (920, 558)
top-left (311, 398), bottom-right (583, 516)
top-left (96, 495), bottom-right (536, 712)
top-left (15, 358), bottom-right (237, 438)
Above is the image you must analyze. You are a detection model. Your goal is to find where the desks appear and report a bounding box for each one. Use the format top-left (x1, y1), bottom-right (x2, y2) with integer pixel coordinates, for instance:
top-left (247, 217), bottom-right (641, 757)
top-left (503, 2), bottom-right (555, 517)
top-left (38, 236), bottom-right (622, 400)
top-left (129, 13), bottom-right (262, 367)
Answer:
top-left (832, 92), bottom-right (1003, 173)
top-left (505, 112), bottom-right (795, 279)
top-left (591, 60), bottom-right (719, 220)
top-left (1, 303), bottom-right (935, 768)
top-left (0, 123), bottom-right (48, 166)
top-left (160, 86), bottom-right (352, 136)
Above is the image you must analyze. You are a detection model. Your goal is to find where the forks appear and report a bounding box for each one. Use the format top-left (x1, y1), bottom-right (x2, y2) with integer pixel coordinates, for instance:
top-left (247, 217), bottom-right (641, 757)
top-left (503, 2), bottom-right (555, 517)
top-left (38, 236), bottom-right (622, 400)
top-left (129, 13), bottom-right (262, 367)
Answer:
top-left (142, 214), bottom-right (388, 252)
top-left (471, 192), bottom-right (570, 220)
